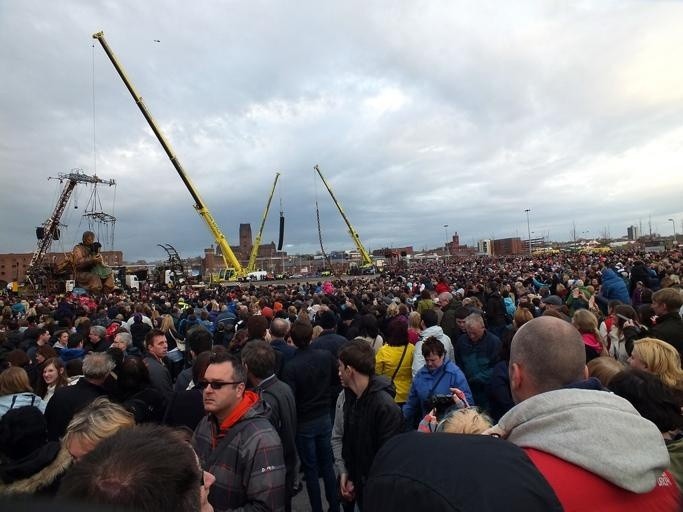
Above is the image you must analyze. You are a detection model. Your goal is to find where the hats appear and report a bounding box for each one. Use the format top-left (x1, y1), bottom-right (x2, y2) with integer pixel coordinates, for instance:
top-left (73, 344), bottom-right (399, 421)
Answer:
top-left (262, 302), bottom-right (284, 320)
top-left (543, 295), bottom-right (563, 305)
top-left (320, 311), bottom-right (337, 329)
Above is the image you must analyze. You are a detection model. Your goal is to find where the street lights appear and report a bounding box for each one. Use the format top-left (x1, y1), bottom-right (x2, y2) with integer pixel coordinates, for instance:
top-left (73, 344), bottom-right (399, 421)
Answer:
top-left (667, 218), bottom-right (676, 241)
top-left (524, 208), bottom-right (531, 255)
top-left (443, 224), bottom-right (449, 256)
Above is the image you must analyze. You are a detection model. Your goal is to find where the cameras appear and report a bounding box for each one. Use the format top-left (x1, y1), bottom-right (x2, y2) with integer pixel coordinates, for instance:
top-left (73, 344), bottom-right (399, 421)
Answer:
top-left (435, 394), bottom-right (456, 423)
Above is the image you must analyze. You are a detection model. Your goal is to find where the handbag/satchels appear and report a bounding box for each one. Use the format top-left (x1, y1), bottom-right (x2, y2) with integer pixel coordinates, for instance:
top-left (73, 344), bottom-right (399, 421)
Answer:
top-left (505, 314), bottom-right (513, 325)
top-left (385, 379), bottom-right (396, 399)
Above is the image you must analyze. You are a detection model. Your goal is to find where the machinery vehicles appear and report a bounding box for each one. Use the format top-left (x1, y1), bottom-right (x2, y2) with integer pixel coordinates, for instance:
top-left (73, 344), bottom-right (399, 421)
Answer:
top-left (245, 170), bottom-right (281, 279)
top-left (91, 28), bottom-right (248, 283)
top-left (311, 163), bottom-right (381, 274)
top-left (11, 166), bottom-right (148, 295)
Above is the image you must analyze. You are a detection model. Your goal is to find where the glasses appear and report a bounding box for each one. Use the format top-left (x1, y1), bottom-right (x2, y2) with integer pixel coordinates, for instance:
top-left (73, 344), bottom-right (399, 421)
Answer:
top-left (198, 380), bottom-right (241, 388)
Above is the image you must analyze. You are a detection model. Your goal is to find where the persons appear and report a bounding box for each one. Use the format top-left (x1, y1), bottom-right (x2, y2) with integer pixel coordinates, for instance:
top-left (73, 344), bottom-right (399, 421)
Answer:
top-left (2, 231), bottom-right (683, 512)
